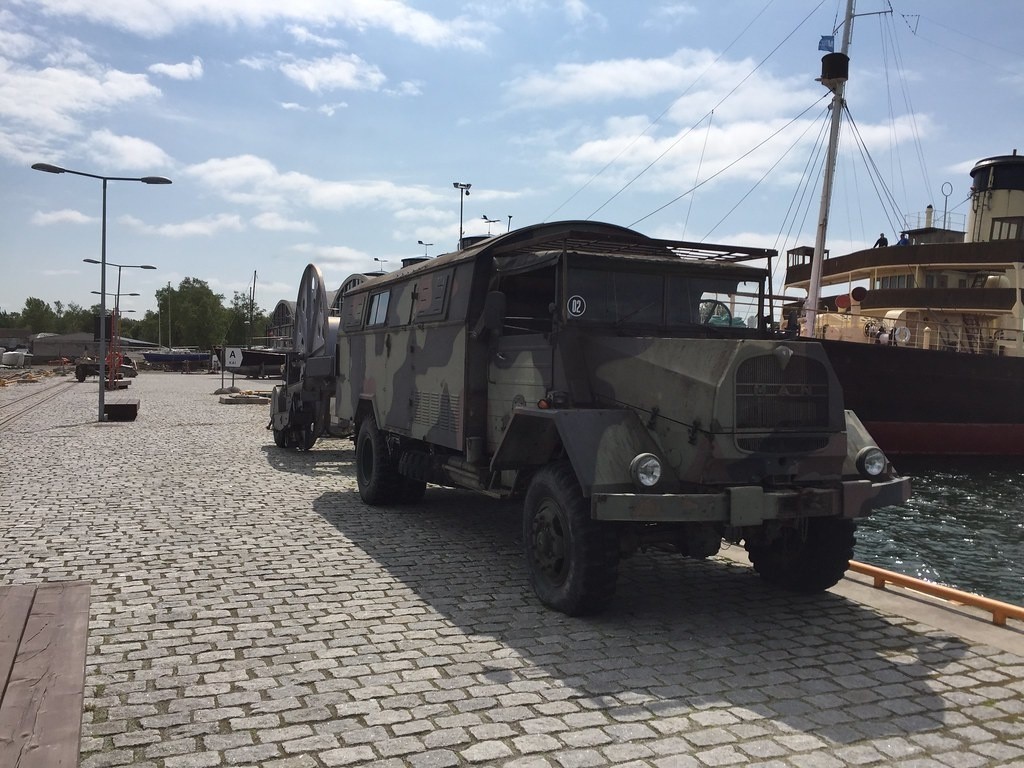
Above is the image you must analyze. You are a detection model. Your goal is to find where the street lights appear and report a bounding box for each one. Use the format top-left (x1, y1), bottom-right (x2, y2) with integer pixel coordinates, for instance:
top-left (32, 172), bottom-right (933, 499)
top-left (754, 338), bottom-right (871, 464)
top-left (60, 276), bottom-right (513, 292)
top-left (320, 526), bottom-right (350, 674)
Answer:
top-left (30, 161), bottom-right (172, 419)
top-left (90, 289), bottom-right (140, 310)
top-left (83, 258), bottom-right (159, 392)
top-left (453, 180), bottom-right (472, 249)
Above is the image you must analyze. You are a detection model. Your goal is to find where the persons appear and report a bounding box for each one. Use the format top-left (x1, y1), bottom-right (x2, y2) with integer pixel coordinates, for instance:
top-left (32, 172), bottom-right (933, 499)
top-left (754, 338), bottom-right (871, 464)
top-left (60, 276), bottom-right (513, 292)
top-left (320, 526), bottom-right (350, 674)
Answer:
top-left (874, 233), bottom-right (888, 248)
top-left (897, 234), bottom-right (909, 245)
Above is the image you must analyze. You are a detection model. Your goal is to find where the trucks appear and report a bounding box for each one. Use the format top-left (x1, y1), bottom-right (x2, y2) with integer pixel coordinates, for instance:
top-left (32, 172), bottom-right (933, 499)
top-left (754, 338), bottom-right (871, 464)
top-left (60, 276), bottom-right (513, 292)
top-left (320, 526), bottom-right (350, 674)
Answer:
top-left (333, 217), bottom-right (913, 604)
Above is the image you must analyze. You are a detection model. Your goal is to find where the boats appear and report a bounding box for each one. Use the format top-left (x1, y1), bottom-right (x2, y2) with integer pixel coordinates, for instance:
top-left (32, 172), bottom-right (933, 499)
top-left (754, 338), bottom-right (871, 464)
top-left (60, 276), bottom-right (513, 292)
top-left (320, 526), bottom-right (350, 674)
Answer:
top-left (559, 1), bottom-right (1024, 461)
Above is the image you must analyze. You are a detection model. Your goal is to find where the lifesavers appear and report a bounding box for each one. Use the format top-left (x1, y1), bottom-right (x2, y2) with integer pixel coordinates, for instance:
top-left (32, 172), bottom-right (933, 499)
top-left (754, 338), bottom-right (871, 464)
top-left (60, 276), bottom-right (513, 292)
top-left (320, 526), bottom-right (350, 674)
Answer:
top-left (894, 326), bottom-right (911, 344)
top-left (106, 352), bottom-right (123, 369)
